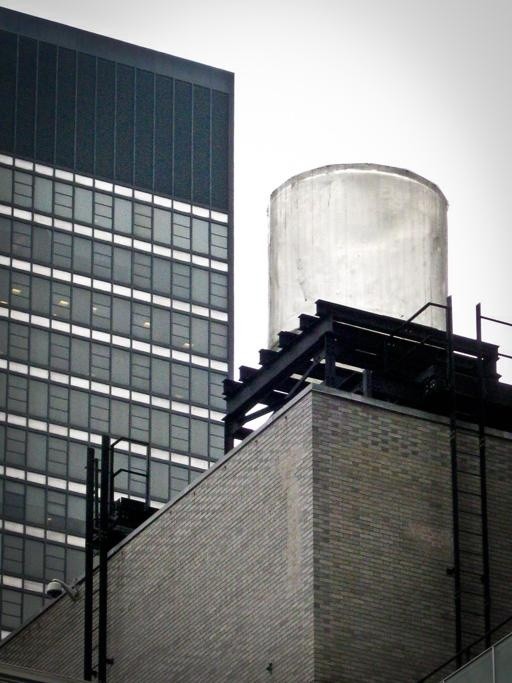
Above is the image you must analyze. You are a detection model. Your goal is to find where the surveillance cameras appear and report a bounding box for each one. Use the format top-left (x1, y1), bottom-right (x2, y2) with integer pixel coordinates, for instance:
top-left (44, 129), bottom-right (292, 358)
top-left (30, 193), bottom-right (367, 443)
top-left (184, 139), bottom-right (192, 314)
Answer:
top-left (46, 581), bottom-right (63, 598)
